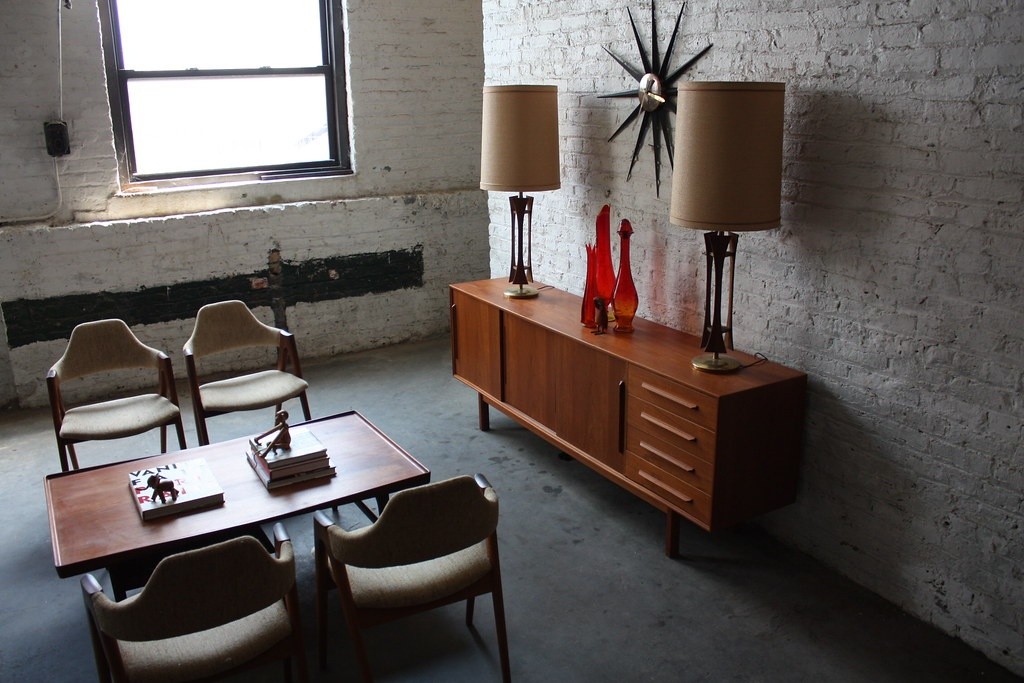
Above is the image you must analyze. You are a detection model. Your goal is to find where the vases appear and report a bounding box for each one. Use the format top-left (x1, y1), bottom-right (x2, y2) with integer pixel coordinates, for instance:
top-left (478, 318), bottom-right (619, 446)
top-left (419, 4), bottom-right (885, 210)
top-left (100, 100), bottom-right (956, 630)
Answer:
top-left (594, 203), bottom-right (616, 323)
top-left (581, 243), bottom-right (599, 329)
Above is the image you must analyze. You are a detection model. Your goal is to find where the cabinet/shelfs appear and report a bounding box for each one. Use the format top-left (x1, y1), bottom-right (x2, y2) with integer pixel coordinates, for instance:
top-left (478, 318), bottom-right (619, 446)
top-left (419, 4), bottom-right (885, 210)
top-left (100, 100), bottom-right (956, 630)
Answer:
top-left (449, 276), bottom-right (809, 558)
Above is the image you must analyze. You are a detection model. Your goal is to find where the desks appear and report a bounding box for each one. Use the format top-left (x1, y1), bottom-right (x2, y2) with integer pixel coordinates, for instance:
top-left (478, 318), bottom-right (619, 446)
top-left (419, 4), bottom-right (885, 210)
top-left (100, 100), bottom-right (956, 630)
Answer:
top-left (41, 408), bottom-right (431, 606)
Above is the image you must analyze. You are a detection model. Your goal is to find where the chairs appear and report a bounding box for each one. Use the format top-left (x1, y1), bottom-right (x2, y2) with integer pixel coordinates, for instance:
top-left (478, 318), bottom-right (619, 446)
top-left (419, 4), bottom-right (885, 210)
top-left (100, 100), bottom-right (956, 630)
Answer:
top-left (312, 471), bottom-right (513, 683)
top-left (182, 301), bottom-right (311, 448)
top-left (78, 520), bottom-right (312, 682)
top-left (46, 319), bottom-right (186, 472)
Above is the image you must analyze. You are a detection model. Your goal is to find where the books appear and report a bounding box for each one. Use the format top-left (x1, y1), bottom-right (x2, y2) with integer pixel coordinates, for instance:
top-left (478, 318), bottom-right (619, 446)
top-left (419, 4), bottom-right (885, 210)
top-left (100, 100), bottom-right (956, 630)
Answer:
top-left (245, 424), bottom-right (339, 491)
top-left (127, 456), bottom-right (225, 525)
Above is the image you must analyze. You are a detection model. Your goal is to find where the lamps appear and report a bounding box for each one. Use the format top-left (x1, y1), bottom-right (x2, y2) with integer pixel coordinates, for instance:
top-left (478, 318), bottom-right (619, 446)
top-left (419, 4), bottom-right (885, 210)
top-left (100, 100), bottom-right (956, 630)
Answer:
top-left (479, 84), bottom-right (561, 299)
top-left (669, 81), bottom-right (786, 375)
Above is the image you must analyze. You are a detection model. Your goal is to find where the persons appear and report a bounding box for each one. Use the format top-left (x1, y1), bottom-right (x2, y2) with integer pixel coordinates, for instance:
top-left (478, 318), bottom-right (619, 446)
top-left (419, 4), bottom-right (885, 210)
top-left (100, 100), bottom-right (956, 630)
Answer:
top-left (254, 410), bottom-right (291, 459)
